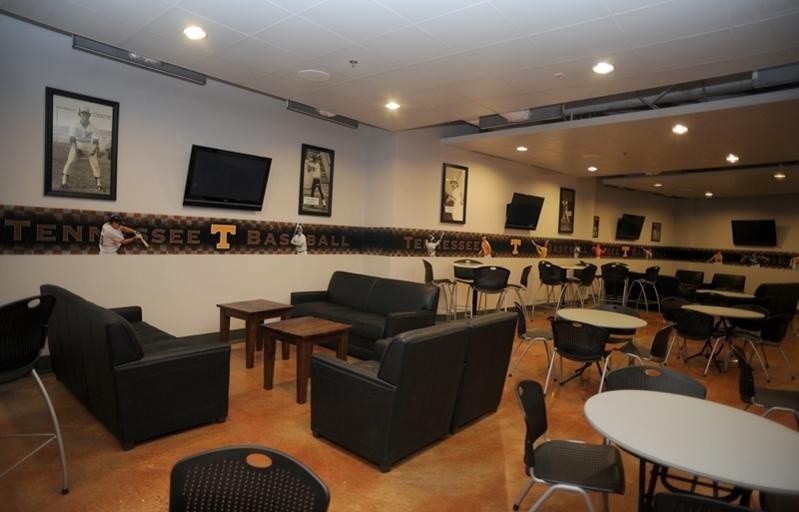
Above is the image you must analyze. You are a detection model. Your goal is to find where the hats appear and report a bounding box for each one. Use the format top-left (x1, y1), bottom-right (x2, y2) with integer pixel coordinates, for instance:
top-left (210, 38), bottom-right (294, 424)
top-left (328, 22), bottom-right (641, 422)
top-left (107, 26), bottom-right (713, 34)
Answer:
top-left (79, 108), bottom-right (90, 115)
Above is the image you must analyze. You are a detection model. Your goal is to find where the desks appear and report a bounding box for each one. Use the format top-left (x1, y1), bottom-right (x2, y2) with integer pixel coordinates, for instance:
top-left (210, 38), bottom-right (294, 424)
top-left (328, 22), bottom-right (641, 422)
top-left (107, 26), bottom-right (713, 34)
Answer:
top-left (262, 316), bottom-right (352, 406)
top-left (582, 389), bottom-right (798, 500)
top-left (216, 298), bottom-right (295, 371)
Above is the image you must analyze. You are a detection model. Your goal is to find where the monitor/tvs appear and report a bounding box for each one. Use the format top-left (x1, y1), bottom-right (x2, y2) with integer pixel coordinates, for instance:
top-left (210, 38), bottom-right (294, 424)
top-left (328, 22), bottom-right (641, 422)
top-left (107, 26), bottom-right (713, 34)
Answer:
top-left (732, 219), bottom-right (776, 246)
top-left (506, 192), bottom-right (544, 230)
top-left (183, 144), bottom-right (272, 211)
top-left (616, 213), bottom-right (645, 240)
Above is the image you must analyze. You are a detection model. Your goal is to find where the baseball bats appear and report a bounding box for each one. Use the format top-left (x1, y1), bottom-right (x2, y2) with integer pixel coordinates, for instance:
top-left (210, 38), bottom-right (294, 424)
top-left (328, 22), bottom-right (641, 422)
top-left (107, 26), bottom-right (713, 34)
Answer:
top-left (303, 153), bottom-right (321, 170)
top-left (439, 232), bottom-right (445, 242)
top-left (294, 222), bottom-right (299, 235)
top-left (141, 239), bottom-right (150, 250)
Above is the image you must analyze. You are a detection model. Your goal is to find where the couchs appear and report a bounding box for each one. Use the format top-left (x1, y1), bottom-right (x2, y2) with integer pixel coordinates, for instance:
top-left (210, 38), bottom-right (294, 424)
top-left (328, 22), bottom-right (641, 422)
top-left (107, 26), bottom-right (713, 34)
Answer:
top-left (307, 310), bottom-right (524, 473)
top-left (287, 272), bottom-right (444, 365)
top-left (39, 275), bottom-right (234, 451)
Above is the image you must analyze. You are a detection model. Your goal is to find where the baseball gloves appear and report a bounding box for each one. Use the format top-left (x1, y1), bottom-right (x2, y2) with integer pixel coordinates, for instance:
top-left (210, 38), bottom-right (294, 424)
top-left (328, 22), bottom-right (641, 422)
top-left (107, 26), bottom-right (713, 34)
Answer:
top-left (76, 148), bottom-right (86, 161)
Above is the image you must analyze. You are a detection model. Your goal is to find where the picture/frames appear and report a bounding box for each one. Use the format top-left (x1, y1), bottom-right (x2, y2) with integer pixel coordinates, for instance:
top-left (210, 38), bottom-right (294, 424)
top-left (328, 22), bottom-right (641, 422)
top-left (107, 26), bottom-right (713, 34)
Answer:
top-left (652, 223), bottom-right (661, 241)
top-left (440, 162), bottom-right (468, 223)
top-left (44, 87), bottom-right (120, 200)
top-left (593, 216), bottom-right (599, 237)
top-left (558, 187), bottom-right (575, 233)
top-left (298, 144), bottom-right (334, 217)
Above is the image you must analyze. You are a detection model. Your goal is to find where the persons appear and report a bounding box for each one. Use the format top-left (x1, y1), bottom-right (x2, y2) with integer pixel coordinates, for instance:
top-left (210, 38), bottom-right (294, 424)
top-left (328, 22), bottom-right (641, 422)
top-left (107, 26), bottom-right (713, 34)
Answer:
top-left (789, 257), bottom-right (799, 270)
top-left (446, 179), bottom-right (463, 221)
top-left (305, 155), bottom-right (327, 208)
top-left (425, 232), bottom-right (445, 257)
top-left (99, 216), bottom-right (142, 255)
top-left (741, 253), bottom-right (769, 268)
top-left (573, 246), bottom-right (587, 258)
top-left (291, 226), bottom-right (307, 255)
top-left (709, 251), bottom-right (723, 265)
top-left (641, 246), bottom-right (653, 259)
top-left (592, 243), bottom-right (606, 257)
top-left (535, 240), bottom-right (549, 258)
top-left (562, 193), bottom-right (570, 224)
top-left (61, 107), bottom-right (104, 192)
top-left (479, 235), bottom-right (492, 257)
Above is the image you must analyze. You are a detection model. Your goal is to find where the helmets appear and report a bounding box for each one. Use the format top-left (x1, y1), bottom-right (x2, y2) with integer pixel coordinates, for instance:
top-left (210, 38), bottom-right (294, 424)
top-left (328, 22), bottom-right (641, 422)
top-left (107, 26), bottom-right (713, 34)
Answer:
top-left (111, 214), bottom-right (126, 227)
top-left (313, 155), bottom-right (320, 159)
top-left (297, 225), bottom-right (303, 231)
top-left (450, 178), bottom-right (459, 186)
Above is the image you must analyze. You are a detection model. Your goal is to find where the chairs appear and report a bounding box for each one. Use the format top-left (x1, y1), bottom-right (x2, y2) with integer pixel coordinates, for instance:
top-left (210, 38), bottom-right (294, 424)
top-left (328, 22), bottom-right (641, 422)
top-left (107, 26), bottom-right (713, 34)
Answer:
top-left (168, 443), bottom-right (332, 511)
top-left (422, 259), bottom-right (799, 399)
top-left (511, 377), bottom-right (626, 510)
top-left (1, 290), bottom-right (69, 497)
top-left (729, 341), bottom-right (798, 427)
top-left (603, 362), bottom-right (714, 448)
top-left (645, 463), bottom-right (749, 512)
